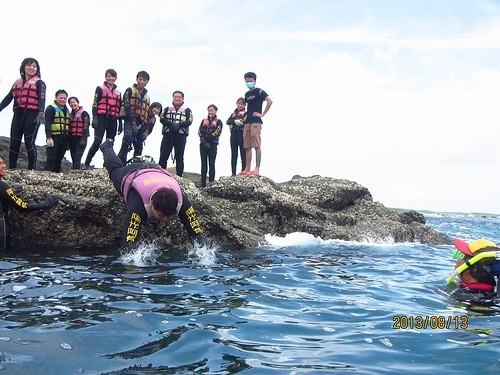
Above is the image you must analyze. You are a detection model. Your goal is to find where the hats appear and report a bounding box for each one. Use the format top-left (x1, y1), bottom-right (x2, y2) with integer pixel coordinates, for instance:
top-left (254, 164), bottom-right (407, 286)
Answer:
top-left (454, 238), bottom-right (496, 256)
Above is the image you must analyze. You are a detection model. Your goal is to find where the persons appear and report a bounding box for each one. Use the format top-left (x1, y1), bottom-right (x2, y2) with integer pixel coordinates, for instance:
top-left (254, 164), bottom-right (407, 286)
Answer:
top-left (226, 71), bottom-right (273, 176)
top-left (118, 71), bottom-right (161, 166)
top-left (99, 139), bottom-right (206, 252)
top-left (451, 238), bottom-right (500, 303)
top-left (158, 91), bottom-right (193, 176)
top-left (68, 97), bottom-right (90, 172)
top-left (0, 58), bottom-right (46, 170)
top-left (82, 69), bottom-right (123, 170)
top-left (45, 90), bottom-right (72, 172)
top-left (197, 104), bottom-right (223, 187)
top-left (0, 156), bottom-right (63, 251)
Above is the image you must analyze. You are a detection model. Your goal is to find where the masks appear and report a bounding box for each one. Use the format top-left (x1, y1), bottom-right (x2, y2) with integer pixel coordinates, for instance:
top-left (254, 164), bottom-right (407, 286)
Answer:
top-left (246, 82), bottom-right (255, 88)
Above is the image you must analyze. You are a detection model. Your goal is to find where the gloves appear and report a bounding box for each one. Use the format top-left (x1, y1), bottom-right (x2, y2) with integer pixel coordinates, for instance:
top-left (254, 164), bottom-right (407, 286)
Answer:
top-left (92, 119), bottom-right (98, 128)
top-left (234, 119), bottom-right (243, 126)
top-left (118, 126), bottom-right (122, 134)
top-left (170, 123), bottom-right (180, 133)
top-left (141, 131), bottom-right (148, 139)
top-left (47, 139), bottom-right (53, 147)
top-left (206, 134), bottom-right (213, 142)
top-left (132, 124), bottom-right (138, 137)
top-left (33, 113), bottom-right (46, 124)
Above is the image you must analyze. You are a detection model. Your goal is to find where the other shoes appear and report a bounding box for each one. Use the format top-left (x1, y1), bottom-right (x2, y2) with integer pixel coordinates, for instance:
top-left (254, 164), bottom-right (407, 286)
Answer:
top-left (99, 138), bottom-right (113, 151)
